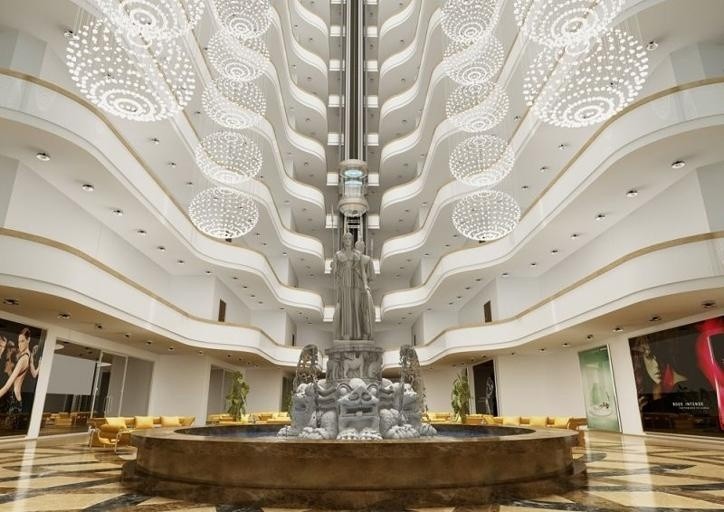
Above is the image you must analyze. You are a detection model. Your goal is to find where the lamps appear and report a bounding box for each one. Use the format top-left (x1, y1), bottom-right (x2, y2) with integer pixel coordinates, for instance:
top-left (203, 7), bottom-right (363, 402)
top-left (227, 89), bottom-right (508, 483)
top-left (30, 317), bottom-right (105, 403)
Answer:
top-left (62, 1), bottom-right (273, 239)
top-left (337, 0), bottom-right (370, 217)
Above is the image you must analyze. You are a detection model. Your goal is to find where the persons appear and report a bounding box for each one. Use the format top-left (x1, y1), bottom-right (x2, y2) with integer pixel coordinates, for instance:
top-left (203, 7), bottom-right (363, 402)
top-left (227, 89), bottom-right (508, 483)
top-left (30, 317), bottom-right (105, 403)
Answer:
top-left (355, 240), bottom-right (375, 339)
top-left (331, 233), bottom-right (370, 339)
top-left (485, 376), bottom-right (494, 414)
top-left (633, 335), bottom-right (688, 430)
top-left (0, 328), bottom-right (42, 429)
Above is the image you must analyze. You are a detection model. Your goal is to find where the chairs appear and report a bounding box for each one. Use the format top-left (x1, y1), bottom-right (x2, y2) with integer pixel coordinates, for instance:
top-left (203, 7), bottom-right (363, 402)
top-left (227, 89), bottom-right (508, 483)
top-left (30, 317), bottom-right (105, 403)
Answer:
top-left (86, 410), bottom-right (292, 456)
top-left (41, 410), bottom-right (97, 428)
top-left (425, 412), bottom-right (589, 450)
